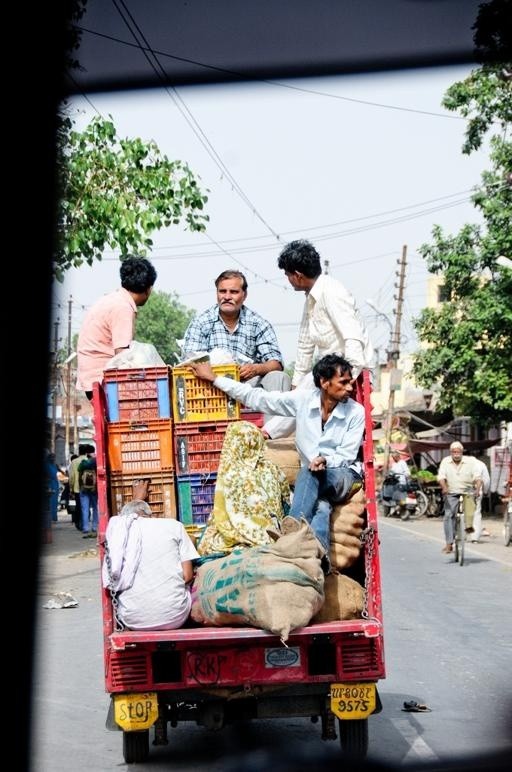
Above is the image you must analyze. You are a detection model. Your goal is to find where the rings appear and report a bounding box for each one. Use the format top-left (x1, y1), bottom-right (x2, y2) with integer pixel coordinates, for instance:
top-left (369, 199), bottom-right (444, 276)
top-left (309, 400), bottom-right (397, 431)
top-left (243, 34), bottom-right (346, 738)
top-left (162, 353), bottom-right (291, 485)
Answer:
top-left (134, 484), bottom-right (139, 487)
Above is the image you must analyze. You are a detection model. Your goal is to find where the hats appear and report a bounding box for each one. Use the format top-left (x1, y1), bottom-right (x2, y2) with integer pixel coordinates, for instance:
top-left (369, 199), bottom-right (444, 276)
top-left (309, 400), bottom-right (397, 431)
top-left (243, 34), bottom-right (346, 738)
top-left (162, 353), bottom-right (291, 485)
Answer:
top-left (449, 440), bottom-right (464, 452)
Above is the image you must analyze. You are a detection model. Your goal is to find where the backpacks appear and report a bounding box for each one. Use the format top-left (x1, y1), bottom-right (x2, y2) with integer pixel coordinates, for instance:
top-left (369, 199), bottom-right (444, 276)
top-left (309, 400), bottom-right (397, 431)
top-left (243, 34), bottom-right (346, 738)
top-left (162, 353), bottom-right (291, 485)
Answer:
top-left (81, 468), bottom-right (96, 493)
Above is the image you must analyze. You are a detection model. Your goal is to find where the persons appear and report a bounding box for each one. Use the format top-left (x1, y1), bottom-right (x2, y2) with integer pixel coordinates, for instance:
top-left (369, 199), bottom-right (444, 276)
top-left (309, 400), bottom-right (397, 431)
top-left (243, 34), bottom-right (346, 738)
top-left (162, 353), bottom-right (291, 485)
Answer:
top-left (46, 443), bottom-right (98, 538)
top-left (187, 353), bottom-right (366, 575)
top-left (99, 478), bottom-right (201, 630)
top-left (435, 440), bottom-right (483, 554)
top-left (76, 257), bottom-right (157, 400)
top-left (182, 270), bottom-right (291, 426)
top-left (260, 237), bottom-right (377, 441)
top-left (198, 420), bottom-right (293, 554)
top-left (390, 452), bottom-right (411, 485)
top-left (460, 456), bottom-right (491, 543)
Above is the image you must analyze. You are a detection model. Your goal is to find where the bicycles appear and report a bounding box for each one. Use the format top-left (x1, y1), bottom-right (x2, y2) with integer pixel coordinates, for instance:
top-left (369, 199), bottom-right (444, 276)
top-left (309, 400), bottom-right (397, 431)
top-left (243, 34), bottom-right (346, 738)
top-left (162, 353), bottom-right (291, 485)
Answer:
top-left (441, 490), bottom-right (478, 567)
top-left (406, 487), bottom-right (430, 519)
top-left (502, 496), bottom-right (512, 547)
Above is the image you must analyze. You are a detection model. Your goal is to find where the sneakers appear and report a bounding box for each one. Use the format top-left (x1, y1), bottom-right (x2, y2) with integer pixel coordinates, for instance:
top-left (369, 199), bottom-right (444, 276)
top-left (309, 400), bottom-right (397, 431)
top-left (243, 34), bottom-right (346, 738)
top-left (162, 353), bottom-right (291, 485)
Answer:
top-left (465, 527), bottom-right (474, 533)
top-left (266, 514), bottom-right (325, 560)
top-left (442, 545), bottom-right (452, 553)
top-left (82, 532), bottom-right (98, 538)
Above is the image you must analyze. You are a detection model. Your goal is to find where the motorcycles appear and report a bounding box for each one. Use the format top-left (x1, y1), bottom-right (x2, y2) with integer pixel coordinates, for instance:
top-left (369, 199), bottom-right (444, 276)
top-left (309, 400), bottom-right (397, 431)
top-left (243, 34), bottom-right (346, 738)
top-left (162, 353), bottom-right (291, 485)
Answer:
top-left (377, 467), bottom-right (420, 521)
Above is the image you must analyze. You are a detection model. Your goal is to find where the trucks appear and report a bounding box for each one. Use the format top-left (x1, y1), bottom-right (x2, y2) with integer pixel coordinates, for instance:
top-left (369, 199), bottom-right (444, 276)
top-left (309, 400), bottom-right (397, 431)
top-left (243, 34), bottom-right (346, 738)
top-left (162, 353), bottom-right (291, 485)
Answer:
top-left (82, 371), bottom-right (390, 768)
